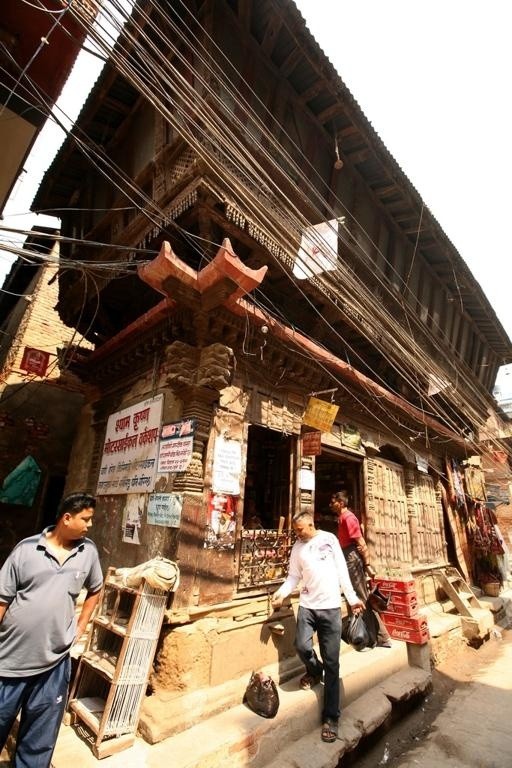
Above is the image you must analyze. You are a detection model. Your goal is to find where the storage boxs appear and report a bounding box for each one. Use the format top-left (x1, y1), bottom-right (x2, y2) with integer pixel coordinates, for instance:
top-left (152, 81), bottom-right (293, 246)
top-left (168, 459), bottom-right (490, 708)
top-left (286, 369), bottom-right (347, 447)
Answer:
top-left (380, 591), bottom-right (417, 605)
top-left (386, 603), bottom-right (418, 617)
top-left (385, 624), bottom-right (430, 645)
top-left (373, 579), bottom-right (416, 594)
top-left (379, 613), bottom-right (428, 631)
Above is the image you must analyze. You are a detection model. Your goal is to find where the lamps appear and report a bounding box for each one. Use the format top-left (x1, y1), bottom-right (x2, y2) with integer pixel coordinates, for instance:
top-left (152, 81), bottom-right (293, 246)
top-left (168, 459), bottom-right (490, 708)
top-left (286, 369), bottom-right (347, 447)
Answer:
top-left (261, 325), bottom-right (269, 334)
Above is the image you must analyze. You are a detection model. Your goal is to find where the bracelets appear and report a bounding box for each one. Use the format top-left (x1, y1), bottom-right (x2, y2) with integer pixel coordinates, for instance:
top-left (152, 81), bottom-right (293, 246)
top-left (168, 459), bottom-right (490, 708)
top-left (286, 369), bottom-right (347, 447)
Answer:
top-left (365, 564), bottom-right (371, 566)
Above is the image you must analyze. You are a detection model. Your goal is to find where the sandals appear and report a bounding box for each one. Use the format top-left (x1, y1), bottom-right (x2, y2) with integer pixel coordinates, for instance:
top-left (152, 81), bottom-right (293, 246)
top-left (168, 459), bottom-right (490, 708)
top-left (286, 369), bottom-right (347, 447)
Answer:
top-left (299, 672), bottom-right (322, 690)
top-left (319, 719), bottom-right (340, 743)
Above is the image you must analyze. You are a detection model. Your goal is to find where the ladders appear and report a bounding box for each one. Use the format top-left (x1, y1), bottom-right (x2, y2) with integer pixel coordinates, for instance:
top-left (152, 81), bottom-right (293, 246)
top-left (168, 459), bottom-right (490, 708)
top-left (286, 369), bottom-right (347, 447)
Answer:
top-left (436, 567), bottom-right (483, 619)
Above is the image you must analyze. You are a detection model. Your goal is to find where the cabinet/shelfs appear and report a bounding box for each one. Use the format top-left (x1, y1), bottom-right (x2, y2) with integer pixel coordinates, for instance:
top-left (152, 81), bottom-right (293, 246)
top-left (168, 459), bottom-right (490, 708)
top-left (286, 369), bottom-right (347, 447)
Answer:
top-left (64, 565), bottom-right (171, 747)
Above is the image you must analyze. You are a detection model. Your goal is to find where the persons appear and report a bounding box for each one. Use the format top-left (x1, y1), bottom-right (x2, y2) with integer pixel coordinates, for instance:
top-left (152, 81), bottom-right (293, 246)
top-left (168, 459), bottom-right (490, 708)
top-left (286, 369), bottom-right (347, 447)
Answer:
top-left (271, 511), bottom-right (366, 743)
top-left (329, 489), bottom-right (392, 647)
top-left (0, 493), bottom-right (104, 768)
